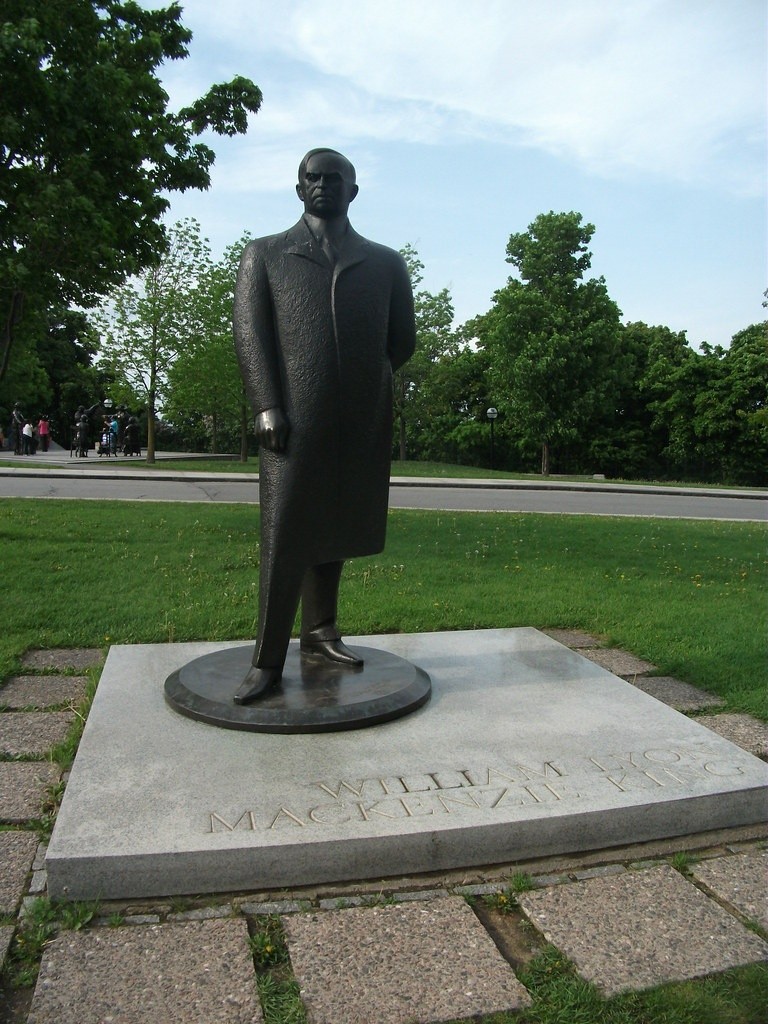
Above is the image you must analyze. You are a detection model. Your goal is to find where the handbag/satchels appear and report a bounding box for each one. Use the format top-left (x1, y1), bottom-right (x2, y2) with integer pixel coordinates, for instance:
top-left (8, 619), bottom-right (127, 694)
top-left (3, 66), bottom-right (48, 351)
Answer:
top-left (22, 435), bottom-right (26, 439)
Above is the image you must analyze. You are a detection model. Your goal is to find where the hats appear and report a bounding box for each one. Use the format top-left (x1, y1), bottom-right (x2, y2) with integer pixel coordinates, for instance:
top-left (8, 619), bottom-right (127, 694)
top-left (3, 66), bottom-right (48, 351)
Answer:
top-left (110, 415), bottom-right (118, 420)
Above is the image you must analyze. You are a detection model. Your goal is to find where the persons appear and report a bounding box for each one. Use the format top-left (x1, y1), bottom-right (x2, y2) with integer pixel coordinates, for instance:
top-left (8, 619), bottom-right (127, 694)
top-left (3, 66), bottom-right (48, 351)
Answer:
top-left (124, 417), bottom-right (141, 457)
top-left (75, 400), bottom-right (102, 457)
top-left (104, 415), bottom-right (119, 457)
top-left (231, 147), bottom-right (417, 704)
top-left (20, 415), bottom-right (50, 455)
top-left (11, 401), bottom-right (30, 455)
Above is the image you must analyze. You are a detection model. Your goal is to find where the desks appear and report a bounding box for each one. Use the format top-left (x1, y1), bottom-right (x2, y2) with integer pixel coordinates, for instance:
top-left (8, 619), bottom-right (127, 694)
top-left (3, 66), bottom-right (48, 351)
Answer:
top-left (99, 431), bottom-right (118, 458)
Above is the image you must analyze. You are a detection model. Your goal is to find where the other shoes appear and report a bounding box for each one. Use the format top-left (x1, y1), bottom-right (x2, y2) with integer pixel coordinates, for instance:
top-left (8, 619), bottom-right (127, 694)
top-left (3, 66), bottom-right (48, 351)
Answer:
top-left (42, 449), bottom-right (47, 451)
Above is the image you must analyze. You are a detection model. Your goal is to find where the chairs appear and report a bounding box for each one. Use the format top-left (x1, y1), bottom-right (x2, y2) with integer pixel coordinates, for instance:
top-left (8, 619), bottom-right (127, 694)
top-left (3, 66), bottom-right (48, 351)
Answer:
top-left (70, 426), bottom-right (88, 458)
